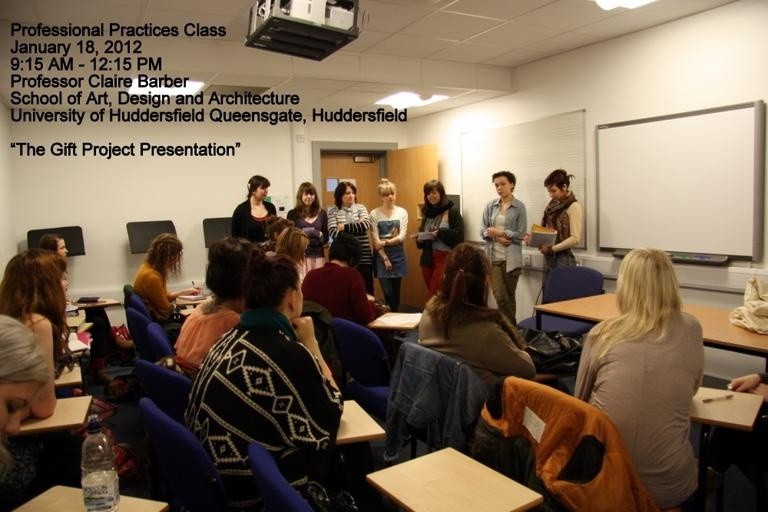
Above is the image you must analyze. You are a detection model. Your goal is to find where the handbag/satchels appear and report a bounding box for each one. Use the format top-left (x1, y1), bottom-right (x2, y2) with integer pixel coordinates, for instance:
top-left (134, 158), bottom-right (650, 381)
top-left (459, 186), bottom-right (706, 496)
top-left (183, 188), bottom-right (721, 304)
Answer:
top-left (520, 326), bottom-right (586, 376)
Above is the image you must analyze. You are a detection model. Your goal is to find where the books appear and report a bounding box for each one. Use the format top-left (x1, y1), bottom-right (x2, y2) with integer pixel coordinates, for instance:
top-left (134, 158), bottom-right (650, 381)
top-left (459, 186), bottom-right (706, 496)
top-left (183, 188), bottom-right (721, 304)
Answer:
top-left (528, 223), bottom-right (558, 248)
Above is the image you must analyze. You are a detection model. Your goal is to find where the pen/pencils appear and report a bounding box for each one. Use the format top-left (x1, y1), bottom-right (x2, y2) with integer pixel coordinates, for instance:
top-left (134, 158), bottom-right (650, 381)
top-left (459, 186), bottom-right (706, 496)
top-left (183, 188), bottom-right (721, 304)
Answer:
top-left (192, 281), bottom-right (194, 289)
top-left (702, 395), bottom-right (733, 402)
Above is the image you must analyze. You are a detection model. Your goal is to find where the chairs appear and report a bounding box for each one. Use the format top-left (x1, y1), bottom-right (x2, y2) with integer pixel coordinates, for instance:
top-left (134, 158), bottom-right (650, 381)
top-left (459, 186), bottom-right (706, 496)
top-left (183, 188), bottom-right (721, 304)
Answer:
top-left (13, 264), bottom-right (766, 512)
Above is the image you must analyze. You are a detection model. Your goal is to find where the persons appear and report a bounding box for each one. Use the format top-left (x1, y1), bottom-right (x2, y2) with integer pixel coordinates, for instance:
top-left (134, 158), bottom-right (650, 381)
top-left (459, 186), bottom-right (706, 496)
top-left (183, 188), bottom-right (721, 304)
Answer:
top-left (704, 351), bottom-right (768, 501)
top-left (524, 168), bottom-right (584, 305)
top-left (370, 178), bottom-right (409, 336)
top-left (287, 182), bottom-right (328, 287)
top-left (481, 171), bottom-right (526, 325)
top-left (231, 175), bottom-right (277, 242)
top-left (573, 250), bottom-right (708, 511)
top-left (417, 179), bottom-right (465, 306)
top-left (324, 182), bottom-right (375, 298)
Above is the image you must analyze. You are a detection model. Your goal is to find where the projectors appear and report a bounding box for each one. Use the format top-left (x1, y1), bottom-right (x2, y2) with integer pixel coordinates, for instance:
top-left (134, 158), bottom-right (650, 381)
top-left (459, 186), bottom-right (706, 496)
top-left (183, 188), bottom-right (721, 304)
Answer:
top-left (244, 0), bottom-right (364, 62)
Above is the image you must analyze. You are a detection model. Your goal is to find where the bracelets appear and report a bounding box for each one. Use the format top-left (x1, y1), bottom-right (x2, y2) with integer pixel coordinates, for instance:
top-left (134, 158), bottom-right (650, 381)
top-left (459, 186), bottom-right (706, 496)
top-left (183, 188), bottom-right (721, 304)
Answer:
top-left (383, 240), bottom-right (388, 246)
top-left (383, 255), bottom-right (388, 260)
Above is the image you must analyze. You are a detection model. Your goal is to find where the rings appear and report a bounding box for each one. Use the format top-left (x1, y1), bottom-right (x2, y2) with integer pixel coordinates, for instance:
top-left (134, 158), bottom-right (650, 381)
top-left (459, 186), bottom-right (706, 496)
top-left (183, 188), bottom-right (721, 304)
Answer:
top-left (725, 381), bottom-right (733, 391)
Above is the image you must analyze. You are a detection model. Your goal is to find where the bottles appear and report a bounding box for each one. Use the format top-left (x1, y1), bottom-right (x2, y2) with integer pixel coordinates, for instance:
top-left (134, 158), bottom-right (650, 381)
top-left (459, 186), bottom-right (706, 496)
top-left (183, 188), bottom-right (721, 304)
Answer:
top-left (79, 416), bottom-right (119, 511)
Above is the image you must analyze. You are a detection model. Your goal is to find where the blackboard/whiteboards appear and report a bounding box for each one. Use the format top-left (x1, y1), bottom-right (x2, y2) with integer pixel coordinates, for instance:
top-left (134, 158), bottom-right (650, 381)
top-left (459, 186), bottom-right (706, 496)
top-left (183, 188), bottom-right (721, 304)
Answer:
top-left (594, 100), bottom-right (765, 265)
